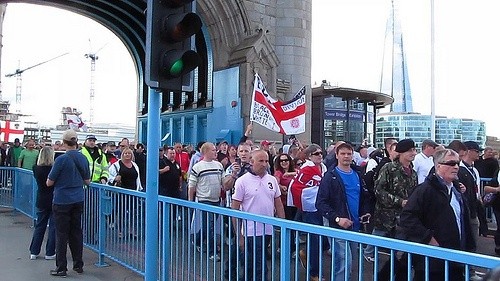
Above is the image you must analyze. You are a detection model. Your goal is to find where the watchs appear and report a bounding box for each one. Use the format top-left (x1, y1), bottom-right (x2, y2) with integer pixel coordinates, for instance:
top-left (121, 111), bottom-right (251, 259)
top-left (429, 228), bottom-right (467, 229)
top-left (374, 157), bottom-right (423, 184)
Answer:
top-left (335, 217), bottom-right (340, 223)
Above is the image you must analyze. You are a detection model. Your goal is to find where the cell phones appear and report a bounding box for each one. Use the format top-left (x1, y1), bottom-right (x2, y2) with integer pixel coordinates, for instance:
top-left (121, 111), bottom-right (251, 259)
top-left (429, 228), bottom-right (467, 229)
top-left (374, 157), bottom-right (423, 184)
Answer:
top-left (235, 158), bottom-right (241, 166)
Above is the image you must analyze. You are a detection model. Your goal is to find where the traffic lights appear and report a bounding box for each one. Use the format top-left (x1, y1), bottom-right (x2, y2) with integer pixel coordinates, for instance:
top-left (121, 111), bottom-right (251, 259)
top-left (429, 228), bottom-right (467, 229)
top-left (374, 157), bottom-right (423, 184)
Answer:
top-left (144, 0), bottom-right (203, 92)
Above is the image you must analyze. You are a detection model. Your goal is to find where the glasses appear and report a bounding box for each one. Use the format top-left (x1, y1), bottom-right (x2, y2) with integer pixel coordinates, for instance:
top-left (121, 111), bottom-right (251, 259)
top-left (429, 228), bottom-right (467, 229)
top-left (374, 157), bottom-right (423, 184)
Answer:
top-left (431, 145), bottom-right (435, 150)
top-left (311, 151), bottom-right (323, 156)
top-left (438, 161), bottom-right (460, 167)
top-left (280, 160), bottom-right (289, 162)
top-left (472, 150), bottom-right (480, 153)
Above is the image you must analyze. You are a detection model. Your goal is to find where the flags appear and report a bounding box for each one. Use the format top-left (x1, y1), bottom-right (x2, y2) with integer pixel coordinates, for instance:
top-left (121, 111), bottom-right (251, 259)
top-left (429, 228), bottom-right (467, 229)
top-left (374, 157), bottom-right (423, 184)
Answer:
top-left (0, 121), bottom-right (24, 144)
top-left (66, 114), bottom-right (84, 130)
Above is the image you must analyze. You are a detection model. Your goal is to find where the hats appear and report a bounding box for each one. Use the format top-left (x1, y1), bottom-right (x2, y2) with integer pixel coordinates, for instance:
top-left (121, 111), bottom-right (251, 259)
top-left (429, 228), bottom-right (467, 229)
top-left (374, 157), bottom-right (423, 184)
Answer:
top-left (85, 135), bottom-right (97, 141)
top-left (394, 138), bottom-right (415, 153)
top-left (464, 141), bottom-right (484, 152)
top-left (422, 137), bottom-right (439, 147)
top-left (107, 140), bottom-right (116, 146)
top-left (62, 128), bottom-right (78, 142)
top-left (14, 138), bottom-right (19, 143)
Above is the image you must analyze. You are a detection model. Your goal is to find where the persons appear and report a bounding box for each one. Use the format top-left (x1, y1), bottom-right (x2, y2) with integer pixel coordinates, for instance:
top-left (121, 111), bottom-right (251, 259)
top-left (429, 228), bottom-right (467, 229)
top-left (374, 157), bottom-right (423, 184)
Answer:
top-left (0, 123), bottom-right (500, 281)
top-left (46, 128), bottom-right (90, 278)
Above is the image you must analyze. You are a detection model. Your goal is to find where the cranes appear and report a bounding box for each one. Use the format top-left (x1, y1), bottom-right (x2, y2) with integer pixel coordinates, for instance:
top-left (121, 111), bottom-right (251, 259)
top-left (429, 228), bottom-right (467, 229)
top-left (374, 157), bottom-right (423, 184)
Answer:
top-left (4, 52), bottom-right (70, 114)
top-left (84, 36), bottom-right (115, 123)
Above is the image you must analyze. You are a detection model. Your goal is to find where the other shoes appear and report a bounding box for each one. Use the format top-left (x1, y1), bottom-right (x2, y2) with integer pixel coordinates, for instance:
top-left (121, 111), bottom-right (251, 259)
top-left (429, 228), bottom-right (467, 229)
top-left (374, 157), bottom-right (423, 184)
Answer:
top-left (268, 244), bottom-right (335, 281)
top-left (44, 254), bottom-right (57, 260)
top-left (49, 268), bottom-right (67, 277)
top-left (31, 253), bottom-right (41, 260)
top-left (190, 240), bottom-right (223, 262)
top-left (107, 221), bottom-right (138, 238)
top-left (363, 253), bottom-right (373, 263)
top-left (74, 269), bottom-right (84, 274)
